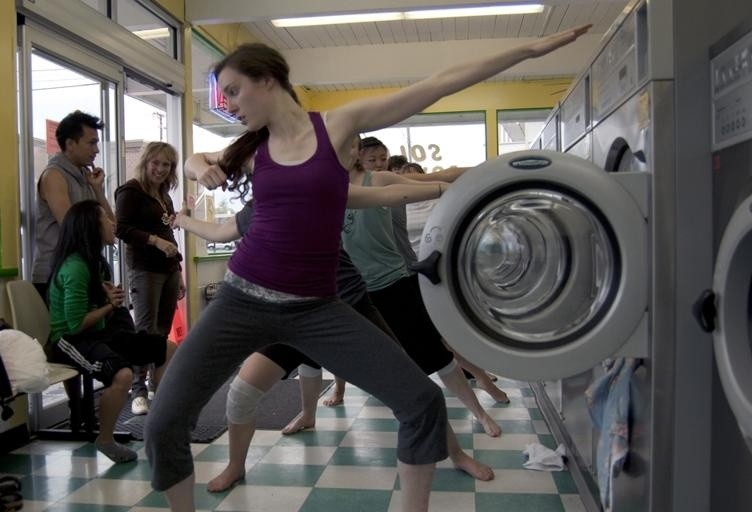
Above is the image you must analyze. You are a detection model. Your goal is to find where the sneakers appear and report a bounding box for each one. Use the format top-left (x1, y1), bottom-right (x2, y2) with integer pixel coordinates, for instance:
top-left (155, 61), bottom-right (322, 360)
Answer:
top-left (131, 391), bottom-right (155, 414)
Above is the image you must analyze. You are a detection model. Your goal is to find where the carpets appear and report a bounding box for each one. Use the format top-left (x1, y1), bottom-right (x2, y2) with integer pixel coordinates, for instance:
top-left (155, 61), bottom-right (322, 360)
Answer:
top-left (92, 353), bottom-right (335, 443)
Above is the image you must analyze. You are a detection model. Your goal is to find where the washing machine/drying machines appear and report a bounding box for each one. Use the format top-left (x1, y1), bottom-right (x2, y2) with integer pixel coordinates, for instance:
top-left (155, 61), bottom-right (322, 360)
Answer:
top-left (416, 1), bottom-right (751, 509)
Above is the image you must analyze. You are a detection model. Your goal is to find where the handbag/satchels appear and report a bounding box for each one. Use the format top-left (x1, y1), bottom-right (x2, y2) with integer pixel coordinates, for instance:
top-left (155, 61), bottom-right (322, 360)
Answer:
top-left (104, 306), bottom-right (136, 334)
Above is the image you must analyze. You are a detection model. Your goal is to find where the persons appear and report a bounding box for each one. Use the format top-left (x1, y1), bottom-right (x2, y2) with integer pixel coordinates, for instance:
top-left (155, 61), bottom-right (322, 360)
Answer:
top-left (31, 109), bottom-right (117, 432)
top-left (160, 137), bottom-right (510, 492)
top-left (143, 23), bottom-right (593, 512)
top-left (114, 142), bottom-right (187, 415)
top-left (43, 200), bottom-right (178, 462)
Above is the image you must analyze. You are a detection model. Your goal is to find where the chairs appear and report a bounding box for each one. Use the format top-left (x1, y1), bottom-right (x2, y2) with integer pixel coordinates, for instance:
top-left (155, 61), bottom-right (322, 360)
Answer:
top-left (7, 278), bottom-right (132, 445)
